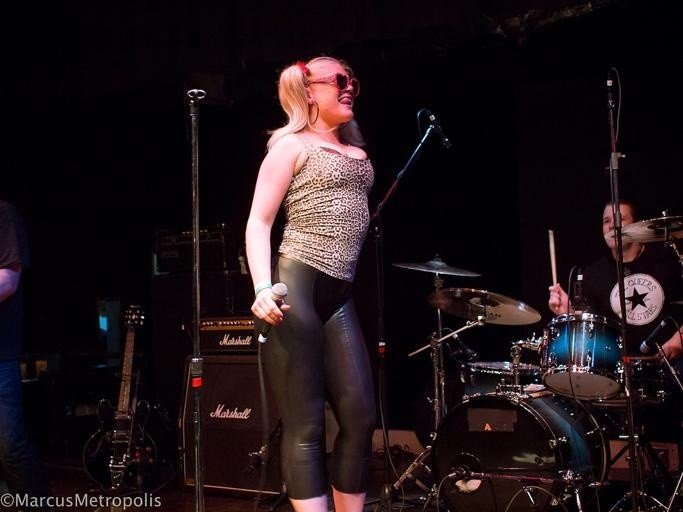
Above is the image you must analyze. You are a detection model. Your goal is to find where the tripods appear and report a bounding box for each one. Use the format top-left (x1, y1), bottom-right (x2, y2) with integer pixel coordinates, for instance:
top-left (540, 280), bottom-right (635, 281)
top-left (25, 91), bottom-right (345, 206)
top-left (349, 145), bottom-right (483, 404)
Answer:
top-left (609, 101), bottom-right (667, 512)
top-left (380, 335), bottom-right (448, 504)
top-left (265, 146), bottom-right (420, 511)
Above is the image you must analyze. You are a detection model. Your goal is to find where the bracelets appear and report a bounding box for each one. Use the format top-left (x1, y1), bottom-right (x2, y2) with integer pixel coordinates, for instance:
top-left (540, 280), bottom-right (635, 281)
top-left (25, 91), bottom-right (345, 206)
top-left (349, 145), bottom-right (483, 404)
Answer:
top-left (255, 284), bottom-right (272, 296)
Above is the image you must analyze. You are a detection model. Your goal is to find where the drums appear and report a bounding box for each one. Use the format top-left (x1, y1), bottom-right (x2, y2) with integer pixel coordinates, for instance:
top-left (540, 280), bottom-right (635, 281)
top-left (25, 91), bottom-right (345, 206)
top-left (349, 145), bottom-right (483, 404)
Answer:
top-left (540, 312), bottom-right (628, 402)
top-left (592, 353), bottom-right (668, 407)
top-left (460, 360), bottom-right (546, 400)
top-left (429, 383), bottom-right (609, 508)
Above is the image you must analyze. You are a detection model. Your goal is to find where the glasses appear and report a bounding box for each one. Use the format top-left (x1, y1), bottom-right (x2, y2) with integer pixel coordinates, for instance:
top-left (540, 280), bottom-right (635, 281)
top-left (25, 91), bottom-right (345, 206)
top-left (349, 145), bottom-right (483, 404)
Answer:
top-left (304, 73), bottom-right (361, 98)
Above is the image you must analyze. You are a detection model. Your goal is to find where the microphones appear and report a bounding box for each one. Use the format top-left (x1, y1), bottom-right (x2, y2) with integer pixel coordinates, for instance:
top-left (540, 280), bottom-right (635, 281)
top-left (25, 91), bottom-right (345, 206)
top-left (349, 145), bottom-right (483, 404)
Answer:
top-left (427, 111), bottom-right (452, 149)
top-left (260, 284), bottom-right (290, 342)
top-left (640, 317), bottom-right (668, 355)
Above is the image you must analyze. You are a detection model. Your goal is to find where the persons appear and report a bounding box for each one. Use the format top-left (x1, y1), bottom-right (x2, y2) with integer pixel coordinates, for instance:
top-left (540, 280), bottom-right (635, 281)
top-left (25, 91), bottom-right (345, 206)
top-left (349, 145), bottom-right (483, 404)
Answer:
top-left (0, 198), bottom-right (51, 512)
top-left (244, 56), bottom-right (376, 512)
top-left (549, 203), bottom-right (683, 362)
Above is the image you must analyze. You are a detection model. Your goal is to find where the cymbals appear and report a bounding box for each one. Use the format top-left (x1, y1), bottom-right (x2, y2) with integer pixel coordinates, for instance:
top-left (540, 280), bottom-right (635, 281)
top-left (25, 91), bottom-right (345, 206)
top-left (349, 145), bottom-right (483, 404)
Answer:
top-left (428, 286), bottom-right (542, 326)
top-left (603, 215), bottom-right (683, 244)
top-left (390, 258), bottom-right (483, 278)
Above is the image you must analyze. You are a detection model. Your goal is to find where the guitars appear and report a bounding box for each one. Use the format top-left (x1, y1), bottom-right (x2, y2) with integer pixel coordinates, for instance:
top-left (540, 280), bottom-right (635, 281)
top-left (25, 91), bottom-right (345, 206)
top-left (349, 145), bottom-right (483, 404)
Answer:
top-left (80, 300), bottom-right (158, 491)
